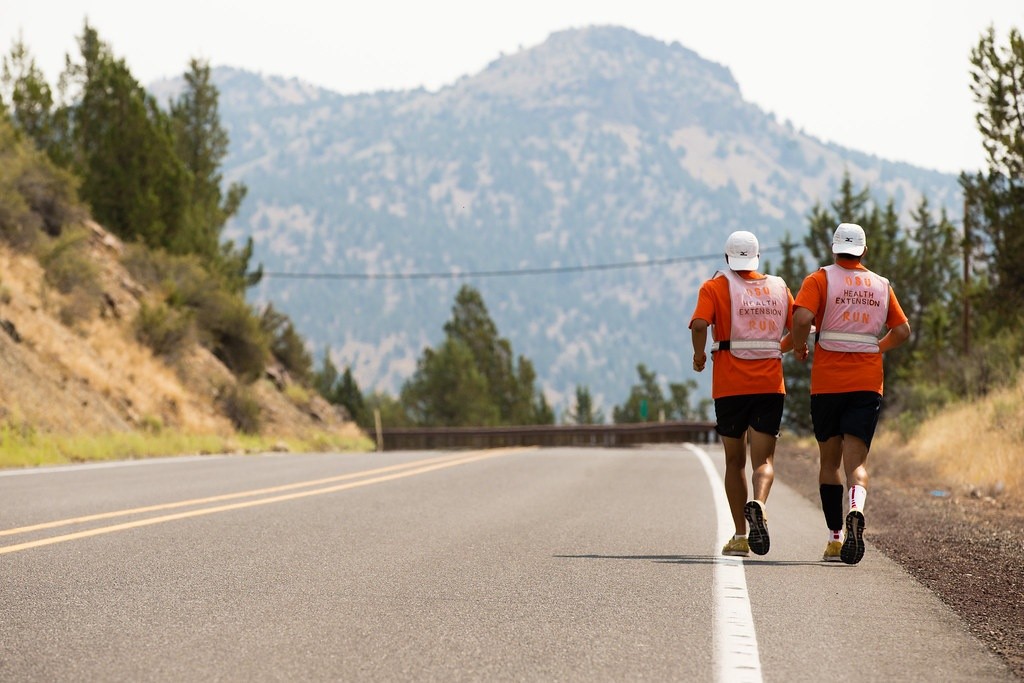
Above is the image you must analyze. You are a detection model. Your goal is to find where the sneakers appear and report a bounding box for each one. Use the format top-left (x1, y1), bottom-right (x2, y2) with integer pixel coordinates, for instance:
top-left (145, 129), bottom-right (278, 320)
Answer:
top-left (722, 536), bottom-right (751, 559)
top-left (822, 530), bottom-right (846, 562)
top-left (743, 500), bottom-right (770, 556)
top-left (840, 508), bottom-right (866, 565)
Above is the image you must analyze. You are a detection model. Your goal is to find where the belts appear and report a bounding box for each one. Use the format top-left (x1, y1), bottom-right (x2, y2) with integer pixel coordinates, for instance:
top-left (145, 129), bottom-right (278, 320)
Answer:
top-left (814, 331), bottom-right (879, 346)
top-left (711, 340), bottom-right (782, 354)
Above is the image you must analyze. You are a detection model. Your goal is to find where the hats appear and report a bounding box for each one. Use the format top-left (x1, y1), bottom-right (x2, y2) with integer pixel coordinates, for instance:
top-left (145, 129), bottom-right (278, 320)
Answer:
top-left (832, 222), bottom-right (867, 256)
top-left (724, 231), bottom-right (760, 271)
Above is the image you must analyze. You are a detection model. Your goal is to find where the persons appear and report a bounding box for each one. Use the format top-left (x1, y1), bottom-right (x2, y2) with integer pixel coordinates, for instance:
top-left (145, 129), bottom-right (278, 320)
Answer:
top-left (689, 231), bottom-right (794, 556)
top-left (791, 223), bottom-right (911, 565)
